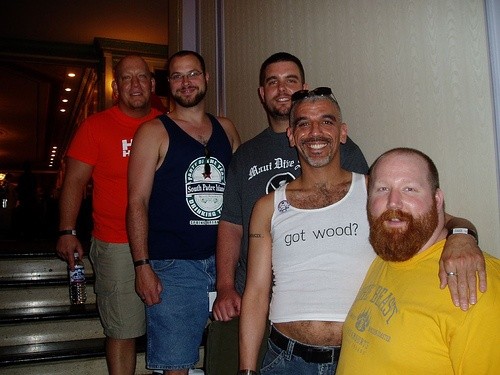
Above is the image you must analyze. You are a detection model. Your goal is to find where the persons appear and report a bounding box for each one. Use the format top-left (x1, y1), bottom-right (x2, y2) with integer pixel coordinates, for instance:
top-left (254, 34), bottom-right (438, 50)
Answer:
top-left (335, 148), bottom-right (499, 375)
top-left (206, 51), bottom-right (370, 374)
top-left (125, 50), bottom-right (243, 374)
top-left (57, 54), bottom-right (163, 374)
top-left (239, 89), bottom-right (487, 374)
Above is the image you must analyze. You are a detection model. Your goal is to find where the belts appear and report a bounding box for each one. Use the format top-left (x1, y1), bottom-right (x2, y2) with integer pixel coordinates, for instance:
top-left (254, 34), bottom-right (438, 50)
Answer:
top-left (269, 326), bottom-right (341, 364)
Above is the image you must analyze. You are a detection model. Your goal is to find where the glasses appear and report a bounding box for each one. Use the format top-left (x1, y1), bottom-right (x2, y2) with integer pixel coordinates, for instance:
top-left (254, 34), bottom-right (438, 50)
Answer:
top-left (169, 70), bottom-right (204, 83)
top-left (289, 87), bottom-right (340, 128)
top-left (204, 149), bottom-right (211, 175)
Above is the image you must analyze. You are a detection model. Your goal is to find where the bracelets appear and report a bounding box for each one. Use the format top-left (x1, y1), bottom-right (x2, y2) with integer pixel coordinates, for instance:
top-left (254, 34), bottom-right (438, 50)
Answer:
top-left (59, 230), bottom-right (76, 236)
top-left (446, 228), bottom-right (478, 241)
top-left (240, 370), bottom-right (254, 375)
top-left (134, 259), bottom-right (149, 267)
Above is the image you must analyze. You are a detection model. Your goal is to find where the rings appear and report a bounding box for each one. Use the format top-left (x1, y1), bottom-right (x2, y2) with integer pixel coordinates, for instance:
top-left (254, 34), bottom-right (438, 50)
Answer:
top-left (448, 272), bottom-right (455, 275)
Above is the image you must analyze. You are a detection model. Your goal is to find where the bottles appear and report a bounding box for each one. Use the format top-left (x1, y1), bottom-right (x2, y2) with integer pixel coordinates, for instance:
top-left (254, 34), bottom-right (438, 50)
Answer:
top-left (67, 252), bottom-right (87, 305)
